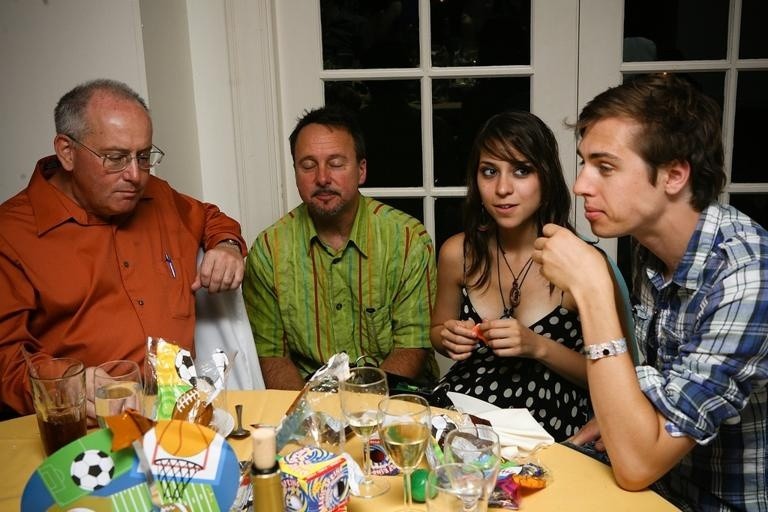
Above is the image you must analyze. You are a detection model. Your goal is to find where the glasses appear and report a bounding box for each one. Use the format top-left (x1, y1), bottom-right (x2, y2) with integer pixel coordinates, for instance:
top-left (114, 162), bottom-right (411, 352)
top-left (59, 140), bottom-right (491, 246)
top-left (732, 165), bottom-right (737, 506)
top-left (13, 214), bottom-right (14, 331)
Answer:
top-left (57, 133), bottom-right (165, 173)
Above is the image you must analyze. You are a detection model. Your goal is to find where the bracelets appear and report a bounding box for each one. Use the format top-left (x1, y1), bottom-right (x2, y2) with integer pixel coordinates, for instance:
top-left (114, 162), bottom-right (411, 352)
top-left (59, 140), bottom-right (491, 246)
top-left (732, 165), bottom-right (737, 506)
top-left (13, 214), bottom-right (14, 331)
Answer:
top-left (584, 338), bottom-right (630, 361)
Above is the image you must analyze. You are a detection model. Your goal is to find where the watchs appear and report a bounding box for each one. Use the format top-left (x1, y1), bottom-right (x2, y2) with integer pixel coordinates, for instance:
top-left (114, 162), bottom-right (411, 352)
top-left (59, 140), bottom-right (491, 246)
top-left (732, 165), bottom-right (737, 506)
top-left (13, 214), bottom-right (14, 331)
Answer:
top-left (226, 238), bottom-right (241, 246)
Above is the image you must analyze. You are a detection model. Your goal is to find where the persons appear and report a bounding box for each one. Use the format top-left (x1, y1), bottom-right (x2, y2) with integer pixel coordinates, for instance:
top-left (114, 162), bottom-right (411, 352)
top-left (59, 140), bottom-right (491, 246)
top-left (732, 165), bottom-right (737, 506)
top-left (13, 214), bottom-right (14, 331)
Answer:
top-left (427, 109), bottom-right (630, 452)
top-left (242, 104), bottom-right (438, 394)
top-left (0, 77), bottom-right (246, 425)
top-left (530, 72), bottom-right (767, 512)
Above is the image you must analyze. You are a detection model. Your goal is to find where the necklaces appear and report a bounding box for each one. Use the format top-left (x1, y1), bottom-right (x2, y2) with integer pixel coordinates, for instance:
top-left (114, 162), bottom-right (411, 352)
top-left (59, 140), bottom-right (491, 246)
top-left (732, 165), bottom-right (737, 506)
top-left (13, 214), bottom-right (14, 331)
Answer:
top-left (496, 229), bottom-right (534, 319)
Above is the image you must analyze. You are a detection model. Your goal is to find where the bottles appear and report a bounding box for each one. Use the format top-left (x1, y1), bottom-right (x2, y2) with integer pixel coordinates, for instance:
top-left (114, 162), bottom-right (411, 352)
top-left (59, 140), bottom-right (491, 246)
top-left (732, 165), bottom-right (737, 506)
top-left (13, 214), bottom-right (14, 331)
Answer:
top-left (250, 427), bottom-right (284, 511)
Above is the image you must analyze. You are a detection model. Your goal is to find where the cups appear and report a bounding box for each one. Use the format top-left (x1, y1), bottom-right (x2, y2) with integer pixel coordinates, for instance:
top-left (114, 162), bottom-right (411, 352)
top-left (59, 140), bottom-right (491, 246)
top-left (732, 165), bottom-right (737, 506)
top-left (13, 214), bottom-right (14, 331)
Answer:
top-left (338, 366), bottom-right (390, 501)
top-left (376, 393), bottom-right (433, 512)
top-left (92, 359), bottom-right (143, 433)
top-left (441, 424), bottom-right (502, 512)
top-left (28, 356), bottom-right (90, 458)
top-left (427, 463), bottom-right (486, 512)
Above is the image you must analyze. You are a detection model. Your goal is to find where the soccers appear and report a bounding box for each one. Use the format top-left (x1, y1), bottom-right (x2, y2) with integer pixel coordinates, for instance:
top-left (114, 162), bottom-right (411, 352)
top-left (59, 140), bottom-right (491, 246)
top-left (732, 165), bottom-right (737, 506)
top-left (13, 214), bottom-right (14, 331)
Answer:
top-left (176, 348), bottom-right (197, 387)
top-left (69, 450), bottom-right (114, 492)
